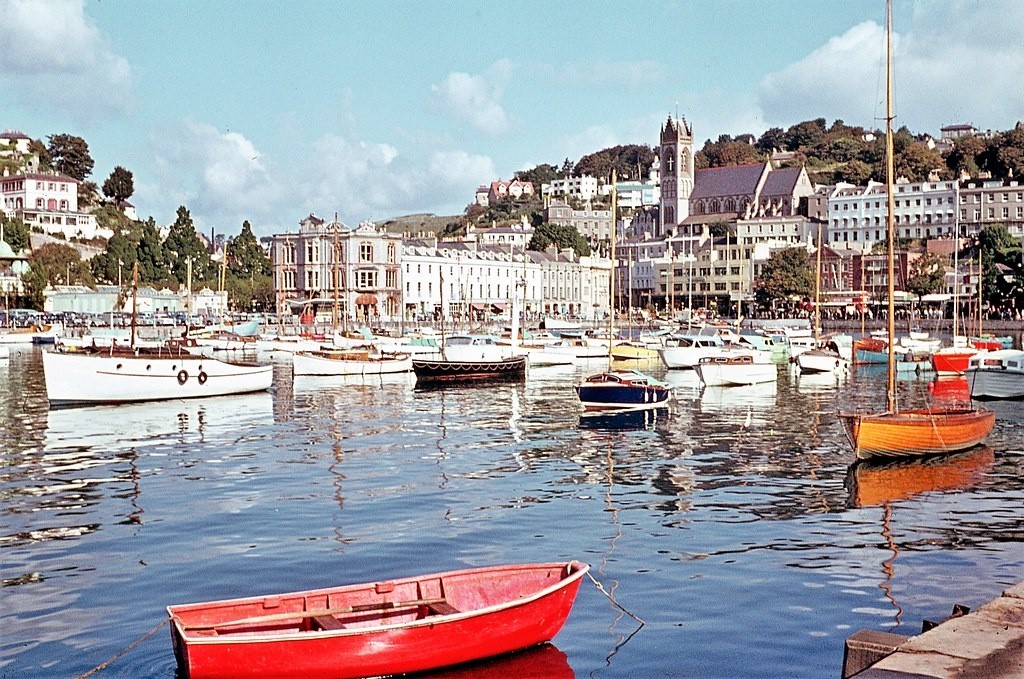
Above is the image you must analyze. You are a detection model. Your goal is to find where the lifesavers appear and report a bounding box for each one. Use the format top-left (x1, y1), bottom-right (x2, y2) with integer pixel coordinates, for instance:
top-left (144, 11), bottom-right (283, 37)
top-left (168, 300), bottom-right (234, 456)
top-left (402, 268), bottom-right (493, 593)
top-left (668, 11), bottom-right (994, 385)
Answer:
top-left (198, 371), bottom-right (208, 384)
top-left (176, 369), bottom-right (189, 383)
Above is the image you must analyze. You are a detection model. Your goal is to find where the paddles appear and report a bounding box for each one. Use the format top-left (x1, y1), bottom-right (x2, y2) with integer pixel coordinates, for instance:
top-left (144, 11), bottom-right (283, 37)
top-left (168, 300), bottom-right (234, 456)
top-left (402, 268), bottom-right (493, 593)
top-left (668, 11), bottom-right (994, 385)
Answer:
top-left (183, 596), bottom-right (448, 630)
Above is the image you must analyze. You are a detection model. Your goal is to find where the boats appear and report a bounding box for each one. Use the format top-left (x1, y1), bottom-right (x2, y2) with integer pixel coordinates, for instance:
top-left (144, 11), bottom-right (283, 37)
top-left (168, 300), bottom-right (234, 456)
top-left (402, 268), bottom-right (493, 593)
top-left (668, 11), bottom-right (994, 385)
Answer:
top-left (834, 406), bottom-right (997, 460)
top-left (657, 334), bottom-right (771, 367)
top-left (963, 350), bottom-right (1024, 399)
top-left (571, 369), bottom-right (674, 408)
top-left (795, 349), bottom-right (852, 375)
top-left (406, 349), bottom-right (529, 392)
top-left (611, 342), bottom-right (659, 359)
top-left (692, 356), bottom-right (777, 386)
top-left (7, 311), bottom-right (1024, 411)
top-left (273, 345), bottom-right (415, 375)
top-left (41, 349), bottom-right (273, 402)
top-left (446, 334), bottom-right (576, 367)
top-left (931, 342), bottom-right (1004, 376)
top-left (164, 559), bottom-right (591, 679)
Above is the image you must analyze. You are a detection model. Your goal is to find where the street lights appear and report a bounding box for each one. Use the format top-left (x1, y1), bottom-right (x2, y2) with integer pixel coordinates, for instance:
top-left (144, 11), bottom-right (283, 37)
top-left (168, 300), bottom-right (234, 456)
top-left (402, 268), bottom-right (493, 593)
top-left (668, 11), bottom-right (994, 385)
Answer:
top-left (183, 253), bottom-right (197, 295)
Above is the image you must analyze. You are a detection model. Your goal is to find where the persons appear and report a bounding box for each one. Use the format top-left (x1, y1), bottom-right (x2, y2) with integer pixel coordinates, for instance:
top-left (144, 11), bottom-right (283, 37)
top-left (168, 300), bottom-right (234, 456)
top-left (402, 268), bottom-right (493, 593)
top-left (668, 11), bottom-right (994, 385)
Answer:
top-left (819, 338), bottom-right (839, 354)
top-left (750, 307), bottom-right (862, 321)
top-left (374, 310), bottom-right (381, 322)
top-left (363, 310), bottom-right (369, 322)
top-left (903, 348), bottom-right (914, 362)
top-left (982, 305), bottom-right (1022, 321)
top-left (882, 305), bottom-right (943, 321)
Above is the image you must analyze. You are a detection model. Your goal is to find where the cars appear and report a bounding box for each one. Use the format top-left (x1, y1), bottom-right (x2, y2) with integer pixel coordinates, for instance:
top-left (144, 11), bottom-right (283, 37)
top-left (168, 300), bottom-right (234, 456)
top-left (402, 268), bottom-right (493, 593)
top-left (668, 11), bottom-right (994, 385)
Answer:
top-left (0, 307), bottom-right (300, 326)
